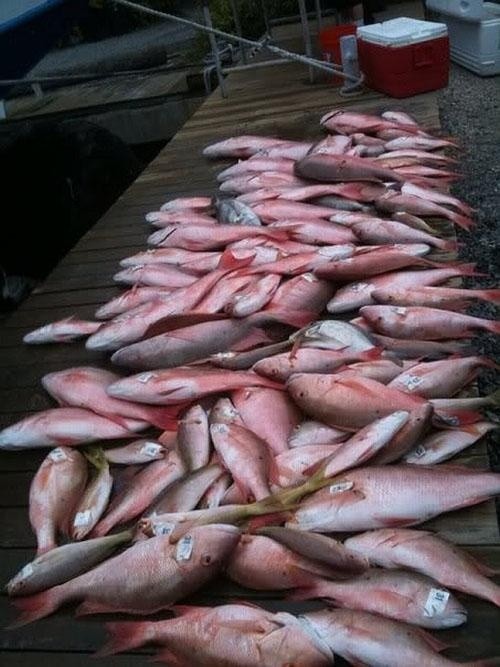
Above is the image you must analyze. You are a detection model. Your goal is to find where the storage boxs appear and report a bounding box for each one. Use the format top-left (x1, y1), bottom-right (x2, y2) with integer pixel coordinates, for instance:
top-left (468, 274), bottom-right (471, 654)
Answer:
top-left (357, 16), bottom-right (452, 99)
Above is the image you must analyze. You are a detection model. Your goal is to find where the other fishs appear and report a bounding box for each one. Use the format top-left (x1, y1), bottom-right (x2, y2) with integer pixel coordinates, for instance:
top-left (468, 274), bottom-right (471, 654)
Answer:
top-left (1, 106), bottom-right (500, 667)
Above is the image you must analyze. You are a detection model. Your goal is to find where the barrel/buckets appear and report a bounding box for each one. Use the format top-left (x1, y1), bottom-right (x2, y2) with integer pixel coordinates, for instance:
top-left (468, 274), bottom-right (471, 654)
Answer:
top-left (319, 24), bottom-right (356, 84)
